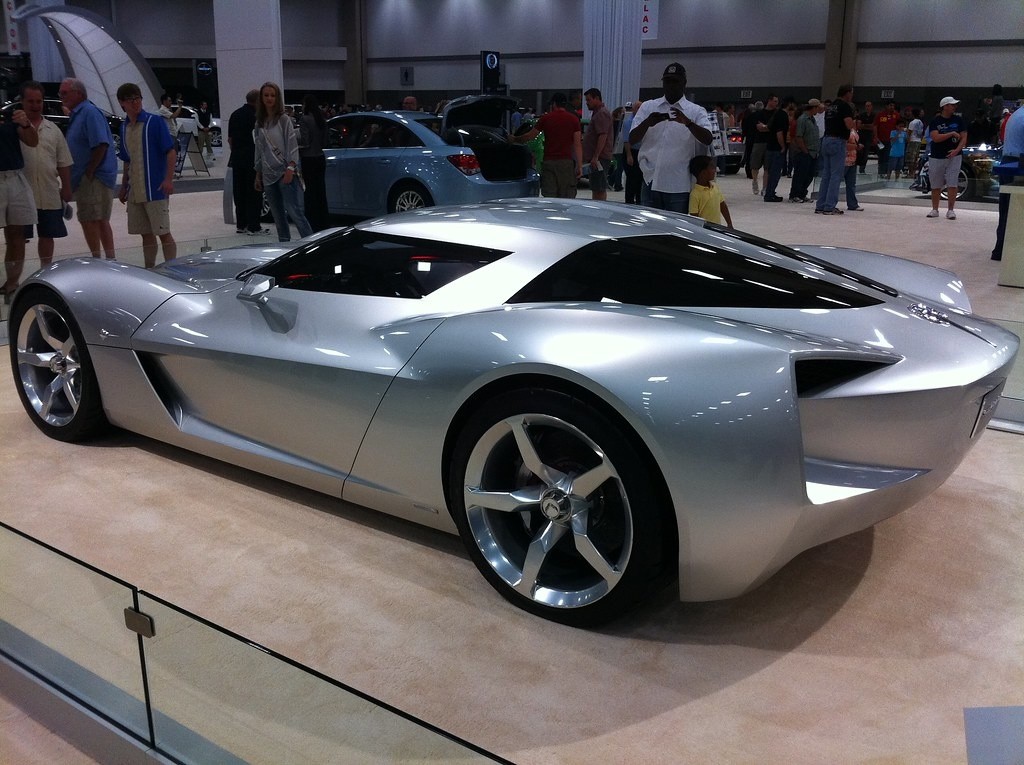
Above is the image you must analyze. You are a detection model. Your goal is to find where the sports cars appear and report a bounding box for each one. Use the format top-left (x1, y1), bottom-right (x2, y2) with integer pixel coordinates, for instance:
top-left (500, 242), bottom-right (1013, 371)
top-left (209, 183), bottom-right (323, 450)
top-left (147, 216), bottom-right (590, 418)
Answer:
top-left (5, 193), bottom-right (1022, 634)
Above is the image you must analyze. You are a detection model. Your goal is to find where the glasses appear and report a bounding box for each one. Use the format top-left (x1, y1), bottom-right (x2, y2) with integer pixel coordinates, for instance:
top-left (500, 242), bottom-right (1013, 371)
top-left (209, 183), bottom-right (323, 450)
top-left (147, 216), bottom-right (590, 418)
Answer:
top-left (58, 90), bottom-right (73, 98)
top-left (124, 96), bottom-right (142, 103)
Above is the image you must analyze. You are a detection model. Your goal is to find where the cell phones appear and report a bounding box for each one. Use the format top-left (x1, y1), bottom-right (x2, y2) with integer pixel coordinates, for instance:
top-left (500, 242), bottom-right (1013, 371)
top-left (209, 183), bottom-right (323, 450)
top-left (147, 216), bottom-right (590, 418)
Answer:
top-left (659, 113), bottom-right (670, 119)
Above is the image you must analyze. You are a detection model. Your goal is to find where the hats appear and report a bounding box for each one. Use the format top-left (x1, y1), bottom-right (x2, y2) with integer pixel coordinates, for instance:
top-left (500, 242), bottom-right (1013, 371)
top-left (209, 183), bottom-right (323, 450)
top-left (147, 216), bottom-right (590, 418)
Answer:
top-left (940, 97), bottom-right (960, 107)
top-left (624, 102), bottom-right (632, 107)
top-left (808, 99), bottom-right (820, 106)
top-left (1002, 109), bottom-right (1009, 114)
top-left (661, 62), bottom-right (686, 80)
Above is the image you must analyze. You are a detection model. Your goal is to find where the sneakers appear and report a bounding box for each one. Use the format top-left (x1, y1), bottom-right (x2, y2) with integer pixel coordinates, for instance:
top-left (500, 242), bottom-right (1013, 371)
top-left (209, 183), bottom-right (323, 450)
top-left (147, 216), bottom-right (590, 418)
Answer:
top-left (946, 209), bottom-right (956, 218)
top-left (247, 226), bottom-right (271, 237)
top-left (236, 227), bottom-right (248, 233)
top-left (926, 210), bottom-right (939, 217)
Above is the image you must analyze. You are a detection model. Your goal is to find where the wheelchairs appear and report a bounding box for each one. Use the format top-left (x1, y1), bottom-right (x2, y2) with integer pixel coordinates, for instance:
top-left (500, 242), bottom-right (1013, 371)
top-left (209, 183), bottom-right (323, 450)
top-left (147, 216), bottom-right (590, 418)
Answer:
top-left (908, 147), bottom-right (980, 202)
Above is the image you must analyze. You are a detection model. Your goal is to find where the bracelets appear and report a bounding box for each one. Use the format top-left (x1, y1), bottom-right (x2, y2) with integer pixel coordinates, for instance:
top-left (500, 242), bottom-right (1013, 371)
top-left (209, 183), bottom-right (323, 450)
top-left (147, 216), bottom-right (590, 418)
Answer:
top-left (687, 119), bottom-right (694, 127)
top-left (22, 125), bottom-right (31, 129)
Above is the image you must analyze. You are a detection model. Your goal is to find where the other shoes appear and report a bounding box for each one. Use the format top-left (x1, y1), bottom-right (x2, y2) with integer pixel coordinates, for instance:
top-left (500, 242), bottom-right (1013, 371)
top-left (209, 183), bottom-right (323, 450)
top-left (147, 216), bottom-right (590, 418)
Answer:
top-left (823, 208), bottom-right (844, 215)
top-left (781, 169), bottom-right (792, 178)
top-left (764, 195), bottom-right (783, 202)
top-left (788, 197), bottom-right (804, 203)
top-left (4, 284), bottom-right (19, 305)
top-left (761, 190), bottom-right (766, 196)
top-left (814, 208), bottom-right (823, 214)
top-left (855, 206), bottom-right (864, 210)
top-left (802, 197), bottom-right (813, 203)
top-left (752, 180), bottom-right (758, 195)
top-left (0, 280), bottom-right (7, 295)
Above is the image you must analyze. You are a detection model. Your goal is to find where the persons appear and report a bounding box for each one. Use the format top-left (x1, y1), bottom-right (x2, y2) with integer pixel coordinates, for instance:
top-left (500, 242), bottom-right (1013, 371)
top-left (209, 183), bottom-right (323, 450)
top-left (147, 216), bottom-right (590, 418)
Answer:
top-left (227, 89), bottom-right (270, 237)
top-left (990, 104), bottom-right (1024, 261)
top-left (582, 88), bottom-right (613, 201)
top-left (195, 101), bottom-right (216, 160)
top-left (117, 83), bottom-right (177, 269)
top-left (507, 93), bottom-right (582, 198)
top-left (157, 93), bottom-right (182, 142)
top-left (402, 96), bottom-right (417, 110)
top-left (285, 93), bottom-right (347, 233)
top-left (688, 155), bottom-right (732, 227)
top-left (58, 77), bottom-right (118, 261)
top-left (612, 85), bottom-right (1011, 216)
top-left (254, 81), bottom-right (314, 242)
top-left (926, 96), bottom-right (968, 219)
top-left (511, 106), bottom-right (531, 134)
top-left (18, 80), bottom-right (74, 266)
top-left (0, 109), bottom-right (38, 295)
top-left (629, 62), bottom-right (713, 214)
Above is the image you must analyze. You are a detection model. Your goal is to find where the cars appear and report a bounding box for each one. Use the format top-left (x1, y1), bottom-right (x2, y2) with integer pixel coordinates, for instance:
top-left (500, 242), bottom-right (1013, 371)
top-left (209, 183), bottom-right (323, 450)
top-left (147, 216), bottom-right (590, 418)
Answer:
top-left (1, 95), bottom-right (129, 159)
top-left (716, 126), bottom-right (752, 178)
top-left (289, 90), bottom-right (544, 228)
top-left (170, 102), bottom-right (222, 149)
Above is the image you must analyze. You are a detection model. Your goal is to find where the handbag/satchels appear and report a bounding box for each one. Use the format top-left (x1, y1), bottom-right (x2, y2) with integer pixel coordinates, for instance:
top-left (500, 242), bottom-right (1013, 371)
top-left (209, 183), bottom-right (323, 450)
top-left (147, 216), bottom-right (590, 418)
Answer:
top-left (611, 131), bottom-right (625, 154)
